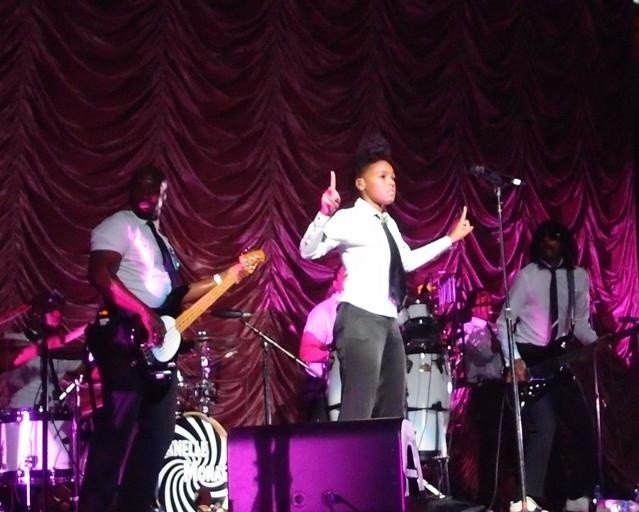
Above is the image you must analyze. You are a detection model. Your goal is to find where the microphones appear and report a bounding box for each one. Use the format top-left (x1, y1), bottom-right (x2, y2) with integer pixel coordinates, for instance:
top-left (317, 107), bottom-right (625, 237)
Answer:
top-left (211, 309), bottom-right (254, 321)
top-left (469, 164), bottom-right (526, 189)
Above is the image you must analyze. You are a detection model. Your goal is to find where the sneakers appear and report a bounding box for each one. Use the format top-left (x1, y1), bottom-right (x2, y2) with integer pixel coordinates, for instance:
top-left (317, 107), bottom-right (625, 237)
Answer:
top-left (566, 496), bottom-right (611, 512)
top-left (508, 496), bottom-right (549, 511)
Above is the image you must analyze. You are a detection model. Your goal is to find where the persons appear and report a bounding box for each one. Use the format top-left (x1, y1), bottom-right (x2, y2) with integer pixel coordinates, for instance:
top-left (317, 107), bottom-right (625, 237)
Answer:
top-left (299, 265), bottom-right (349, 422)
top-left (298, 159), bottom-right (474, 422)
top-left (1, 288), bottom-right (82, 411)
top-left (495, 218), bottom-right (613, 510)
top-left (78, 166), bottom-right (251, 511)
top-left (456, 285), bottom-right (528, 511)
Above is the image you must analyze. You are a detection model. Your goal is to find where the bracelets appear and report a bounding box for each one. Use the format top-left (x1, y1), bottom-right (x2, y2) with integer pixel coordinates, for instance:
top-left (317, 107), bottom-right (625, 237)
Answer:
top-left (212, 273), bottom-right (222, 285)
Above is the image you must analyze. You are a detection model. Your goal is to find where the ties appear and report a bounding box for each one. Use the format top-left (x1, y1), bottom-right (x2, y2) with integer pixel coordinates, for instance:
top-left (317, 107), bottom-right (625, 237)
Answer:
top-left (376, 215), bottom-right (405, 306)
top-left (545, 266), bottom-right (559, 345)
top-left (146, 220), bottom-right (177, 290)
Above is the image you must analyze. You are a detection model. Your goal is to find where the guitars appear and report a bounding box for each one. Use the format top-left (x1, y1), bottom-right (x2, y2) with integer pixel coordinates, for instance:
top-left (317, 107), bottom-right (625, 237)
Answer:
top-left (505, 326), bottom-right (637, 412)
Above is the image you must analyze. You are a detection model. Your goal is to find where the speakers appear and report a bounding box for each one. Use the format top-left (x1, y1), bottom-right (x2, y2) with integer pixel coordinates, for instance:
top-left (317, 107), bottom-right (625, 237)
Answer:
top-left (227, 417), bottom-right (426, 512)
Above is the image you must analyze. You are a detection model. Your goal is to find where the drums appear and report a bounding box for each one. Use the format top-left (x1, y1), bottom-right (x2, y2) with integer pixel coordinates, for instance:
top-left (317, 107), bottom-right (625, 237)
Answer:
top-left (404, 341), bottom-right (454, 462)
top-left (398, 300), bottom-right (432, 330)
top-left (0, 407), bottom-right (74, 485)
top-left (153, 409), bottom-right (230, 508)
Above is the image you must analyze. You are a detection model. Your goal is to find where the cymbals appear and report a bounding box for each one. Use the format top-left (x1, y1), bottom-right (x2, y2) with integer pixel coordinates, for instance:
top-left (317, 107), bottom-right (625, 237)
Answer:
top-left (0, 304), bottom-right (32, 324)
top-left (179, 337), bottom-right (240, 350)
